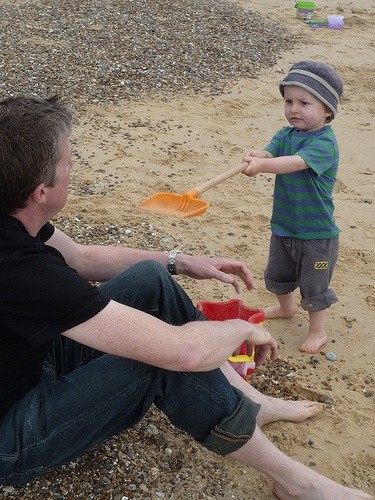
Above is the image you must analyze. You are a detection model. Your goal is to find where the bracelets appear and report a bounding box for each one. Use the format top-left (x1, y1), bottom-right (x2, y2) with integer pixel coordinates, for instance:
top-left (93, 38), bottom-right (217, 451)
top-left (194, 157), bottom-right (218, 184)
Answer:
top-left (167, 250), bottom-right (178, 275)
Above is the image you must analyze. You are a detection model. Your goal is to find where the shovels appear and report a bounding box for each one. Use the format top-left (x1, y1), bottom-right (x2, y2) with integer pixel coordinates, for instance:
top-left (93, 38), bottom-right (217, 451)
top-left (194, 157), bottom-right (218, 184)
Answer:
top-left (137, 151), bottom-right (272, 216)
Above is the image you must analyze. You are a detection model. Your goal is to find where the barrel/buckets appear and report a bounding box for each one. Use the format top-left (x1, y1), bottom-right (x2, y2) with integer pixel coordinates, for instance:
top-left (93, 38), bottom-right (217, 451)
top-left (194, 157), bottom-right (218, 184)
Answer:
top-left (197, 299), bottom-right (264, 381)
top-left (328, 15), bottom-right (344, 30)
top-left (295, 0), bottom-right (315, 19)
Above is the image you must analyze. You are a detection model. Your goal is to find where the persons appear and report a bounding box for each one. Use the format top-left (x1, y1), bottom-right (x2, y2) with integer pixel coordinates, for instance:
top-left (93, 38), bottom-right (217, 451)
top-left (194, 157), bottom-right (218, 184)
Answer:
top-left (244, 60), bottom-right (341, 353)
top-left (1, 96), bottom-right (374, 499)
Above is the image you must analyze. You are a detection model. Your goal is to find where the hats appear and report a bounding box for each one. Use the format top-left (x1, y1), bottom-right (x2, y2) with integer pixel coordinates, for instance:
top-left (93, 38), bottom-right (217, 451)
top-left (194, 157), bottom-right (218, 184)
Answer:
top-left (278, 59), bottom-right (343, 123)
